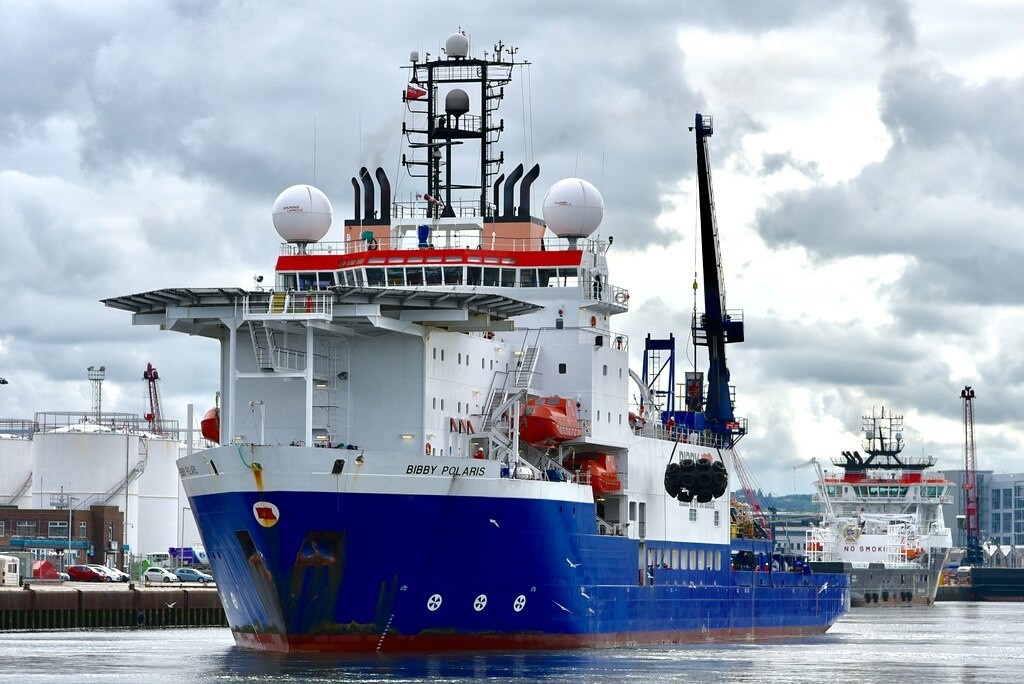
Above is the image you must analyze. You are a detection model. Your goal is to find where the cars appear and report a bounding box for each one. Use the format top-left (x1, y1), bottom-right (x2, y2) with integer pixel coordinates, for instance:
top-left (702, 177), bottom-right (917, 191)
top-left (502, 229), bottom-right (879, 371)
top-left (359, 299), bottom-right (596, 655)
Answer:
top-left (174, 567), bottom-right (214, 583)
top-left (143, 566), bottom-right (178, 583)
top-left (64, 563), bottom-right (131, 583)
top-left (57, 572), bottom-right (70, 581)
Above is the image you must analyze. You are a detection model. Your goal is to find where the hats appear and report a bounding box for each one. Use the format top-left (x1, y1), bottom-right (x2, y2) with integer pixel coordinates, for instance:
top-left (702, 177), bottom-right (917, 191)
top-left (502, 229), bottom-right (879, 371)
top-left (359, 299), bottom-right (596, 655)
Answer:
top-left (479, 447), bottom-right (484, 452)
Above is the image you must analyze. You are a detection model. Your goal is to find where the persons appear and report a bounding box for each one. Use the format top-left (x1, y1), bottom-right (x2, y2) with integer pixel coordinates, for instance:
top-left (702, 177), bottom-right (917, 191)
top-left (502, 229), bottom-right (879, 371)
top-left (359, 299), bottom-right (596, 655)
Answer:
top-left (788, 564), bottom-right (793, 572)
top-left (730, 562), bottom-right (736, 571)
top-left (764, 562), bottom-right (769, 571)
top-left (474, 447), bottom-right (484, 459)
top-left (500, 459), bottom-right (507, 478)
top-left (666, 417), bottom-right (675, 440)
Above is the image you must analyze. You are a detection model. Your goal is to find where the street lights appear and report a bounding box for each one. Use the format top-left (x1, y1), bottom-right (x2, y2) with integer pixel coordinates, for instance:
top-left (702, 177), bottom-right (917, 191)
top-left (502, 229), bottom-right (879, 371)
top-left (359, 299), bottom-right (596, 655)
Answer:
top-left (181, 507), bottom-right (190, 569)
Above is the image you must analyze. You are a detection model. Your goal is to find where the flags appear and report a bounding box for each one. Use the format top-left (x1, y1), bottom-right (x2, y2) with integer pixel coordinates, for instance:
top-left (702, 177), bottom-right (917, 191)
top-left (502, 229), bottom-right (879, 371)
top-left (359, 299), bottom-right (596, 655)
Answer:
top-left (407, 84), bottom-right (426, 100)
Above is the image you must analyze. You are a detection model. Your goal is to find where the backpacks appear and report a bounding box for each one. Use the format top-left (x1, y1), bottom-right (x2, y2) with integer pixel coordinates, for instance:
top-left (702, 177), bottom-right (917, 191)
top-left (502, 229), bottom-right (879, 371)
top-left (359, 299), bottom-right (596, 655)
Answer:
top-left (474, 454), bottom-right (479, 458)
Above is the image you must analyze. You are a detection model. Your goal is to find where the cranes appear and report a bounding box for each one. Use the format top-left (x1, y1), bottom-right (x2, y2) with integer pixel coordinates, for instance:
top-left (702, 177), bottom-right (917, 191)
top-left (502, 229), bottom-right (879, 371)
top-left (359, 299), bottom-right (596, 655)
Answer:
top-left (141, 361), bottom-right (168, 436)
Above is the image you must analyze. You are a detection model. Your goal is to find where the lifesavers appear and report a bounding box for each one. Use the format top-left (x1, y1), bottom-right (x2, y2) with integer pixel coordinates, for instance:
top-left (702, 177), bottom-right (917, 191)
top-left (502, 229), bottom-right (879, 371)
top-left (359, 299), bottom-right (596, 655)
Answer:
top-left (615, 293), bottom-right (627, 303)
top-left (425, 442), bottom-right (432, 456)
top-left (592, 315), bottom-right (596, 328)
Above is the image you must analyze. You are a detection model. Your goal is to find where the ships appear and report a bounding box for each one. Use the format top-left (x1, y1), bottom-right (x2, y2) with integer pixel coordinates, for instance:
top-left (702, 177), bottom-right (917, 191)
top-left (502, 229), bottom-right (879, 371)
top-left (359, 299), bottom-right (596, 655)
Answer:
top-left (96, 21), bottom-right (857, 655)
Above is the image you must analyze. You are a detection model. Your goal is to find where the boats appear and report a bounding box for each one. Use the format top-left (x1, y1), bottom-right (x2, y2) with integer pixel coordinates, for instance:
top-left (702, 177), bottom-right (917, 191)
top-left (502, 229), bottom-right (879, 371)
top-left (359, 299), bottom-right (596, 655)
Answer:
top-left (504, 395), bottom-right (582, 445)
top-left (790, 404), bottom-right (955, 608)
top-left (198, 405), bottom-right (221, 444)
top-left (560, 450), bottom-right (626, 494)
